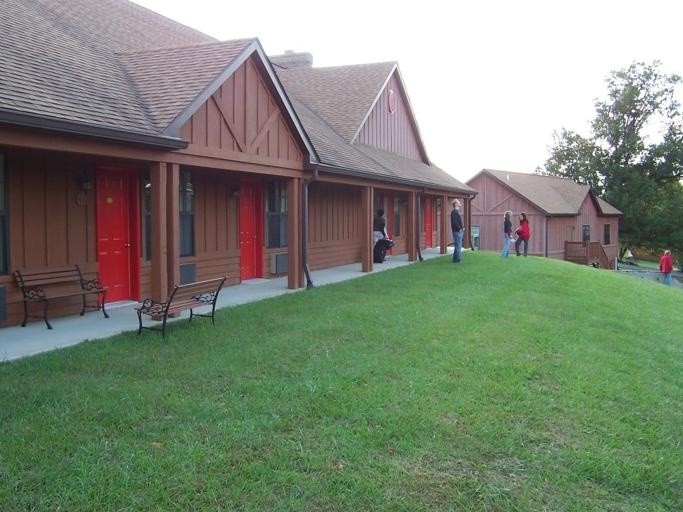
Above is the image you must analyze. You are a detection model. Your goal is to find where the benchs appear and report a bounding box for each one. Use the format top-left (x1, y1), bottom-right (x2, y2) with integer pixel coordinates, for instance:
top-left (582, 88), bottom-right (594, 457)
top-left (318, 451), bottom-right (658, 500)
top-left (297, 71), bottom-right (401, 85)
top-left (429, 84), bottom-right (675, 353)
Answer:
top-left (135, 272), bottom-right (231, 340)
top-left (12, 264), bottom-right (110, 328)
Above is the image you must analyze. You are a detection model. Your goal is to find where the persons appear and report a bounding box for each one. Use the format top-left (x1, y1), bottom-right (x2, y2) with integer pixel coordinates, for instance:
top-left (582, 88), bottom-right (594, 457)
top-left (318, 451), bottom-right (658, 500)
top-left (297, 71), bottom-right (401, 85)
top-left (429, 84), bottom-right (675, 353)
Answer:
top-left (450, 198), bottom-right (465, 264)
top-left (373, 209), bottom-right (389, 247)
top-left (500, 210), bottom-right (531, 258)
top-left (659, 250), bottom-right (673, 286)
top-left (624, 246), bottom-right (642, 263)
